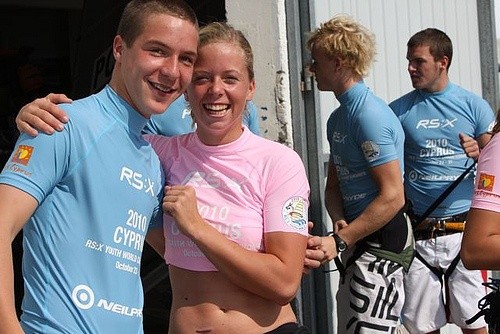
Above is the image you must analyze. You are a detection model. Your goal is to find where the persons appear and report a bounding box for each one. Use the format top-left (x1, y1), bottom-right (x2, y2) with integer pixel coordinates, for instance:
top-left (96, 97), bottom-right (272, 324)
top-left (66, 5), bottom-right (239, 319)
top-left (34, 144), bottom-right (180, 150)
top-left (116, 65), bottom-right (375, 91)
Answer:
top-left (306, 14), bottom-right (407, 333)
top-left (0, 0), bottom-right (324, 333)
top-left (148, 89), bottom-right (263, 166)
top-left (461, 114), bottom-right (500, 333)
top-left (15, 20), bottom-right (313, 334)
top-left (389, 28), bottom-right (499, 333)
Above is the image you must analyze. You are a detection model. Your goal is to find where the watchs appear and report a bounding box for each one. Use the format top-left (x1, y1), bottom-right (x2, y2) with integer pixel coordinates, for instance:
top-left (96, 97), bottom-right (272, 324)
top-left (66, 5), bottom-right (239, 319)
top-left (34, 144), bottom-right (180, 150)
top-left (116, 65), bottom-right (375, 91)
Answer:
top-left (333, 232), bottom-right (349, 252)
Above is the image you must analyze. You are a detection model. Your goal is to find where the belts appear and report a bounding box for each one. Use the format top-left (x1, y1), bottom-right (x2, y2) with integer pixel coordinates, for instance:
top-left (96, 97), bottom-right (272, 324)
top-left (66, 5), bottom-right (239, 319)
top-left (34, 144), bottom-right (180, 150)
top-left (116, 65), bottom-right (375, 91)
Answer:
top-left (414, 217), bottom-right (467, 232)
